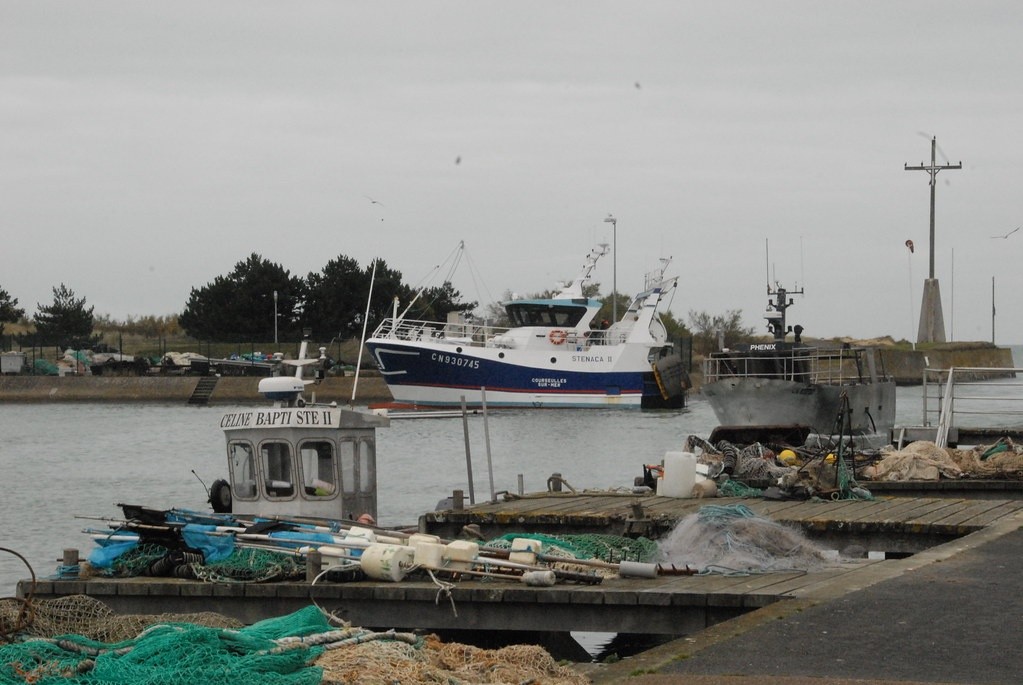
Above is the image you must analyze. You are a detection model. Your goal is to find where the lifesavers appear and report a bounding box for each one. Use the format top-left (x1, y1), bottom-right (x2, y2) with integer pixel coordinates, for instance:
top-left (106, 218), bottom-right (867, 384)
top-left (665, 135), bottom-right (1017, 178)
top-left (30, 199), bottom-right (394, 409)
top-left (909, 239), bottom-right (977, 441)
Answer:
top-left (549, 329), bottom-right (565, 345)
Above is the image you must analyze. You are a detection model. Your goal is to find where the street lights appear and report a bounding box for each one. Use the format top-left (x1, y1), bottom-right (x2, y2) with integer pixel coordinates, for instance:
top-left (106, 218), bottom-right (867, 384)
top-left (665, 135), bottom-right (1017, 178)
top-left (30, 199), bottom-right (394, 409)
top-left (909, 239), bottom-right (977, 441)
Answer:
top-left (604, 218), bottom-right (617, 325)
top-left (273, 290), bottom-right (278, 343)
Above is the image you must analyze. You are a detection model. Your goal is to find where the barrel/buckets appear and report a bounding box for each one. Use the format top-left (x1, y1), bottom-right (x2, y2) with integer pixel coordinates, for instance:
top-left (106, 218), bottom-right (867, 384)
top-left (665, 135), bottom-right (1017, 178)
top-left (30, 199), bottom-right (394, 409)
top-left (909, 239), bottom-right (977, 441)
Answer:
top-left (665, 452), bottom-right (696, 499)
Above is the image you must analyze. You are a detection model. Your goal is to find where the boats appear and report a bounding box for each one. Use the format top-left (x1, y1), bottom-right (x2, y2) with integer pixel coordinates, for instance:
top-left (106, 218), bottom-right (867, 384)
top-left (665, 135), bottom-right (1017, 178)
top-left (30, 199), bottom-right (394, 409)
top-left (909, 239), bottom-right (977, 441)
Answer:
top-left (365, 241), bottom-right (680, 409)
top-left (702, 347), bottom-right (896, 434)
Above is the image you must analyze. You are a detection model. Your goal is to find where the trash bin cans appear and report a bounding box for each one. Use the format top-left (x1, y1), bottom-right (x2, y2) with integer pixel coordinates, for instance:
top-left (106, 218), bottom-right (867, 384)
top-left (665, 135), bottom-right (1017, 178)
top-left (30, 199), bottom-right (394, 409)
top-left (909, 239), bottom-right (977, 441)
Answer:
top-left (0, 351), bottom-right (26, 376)
